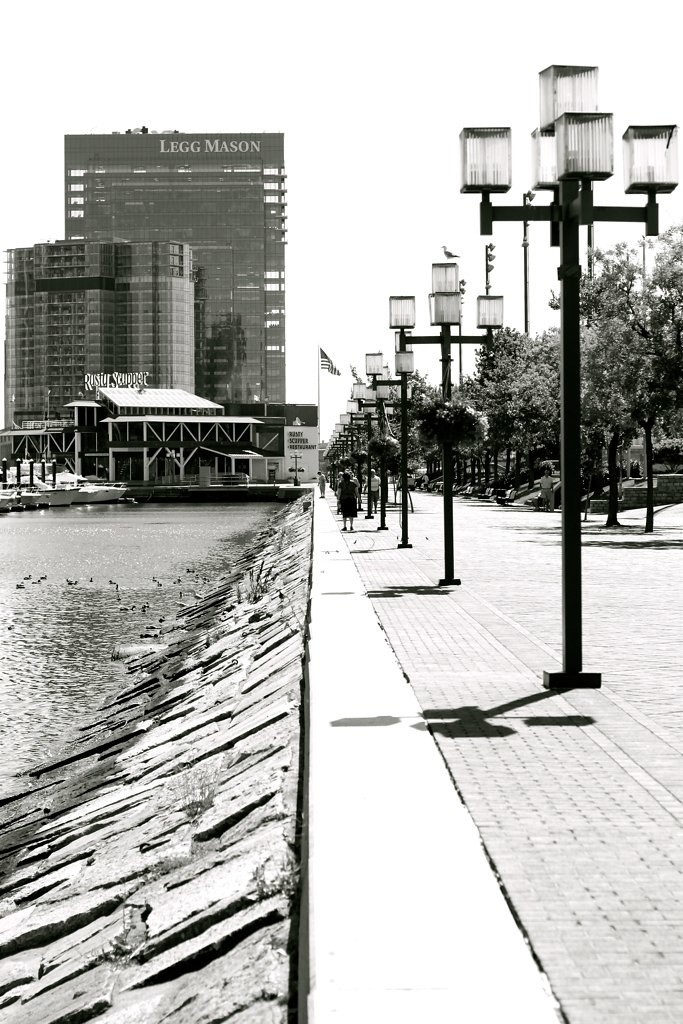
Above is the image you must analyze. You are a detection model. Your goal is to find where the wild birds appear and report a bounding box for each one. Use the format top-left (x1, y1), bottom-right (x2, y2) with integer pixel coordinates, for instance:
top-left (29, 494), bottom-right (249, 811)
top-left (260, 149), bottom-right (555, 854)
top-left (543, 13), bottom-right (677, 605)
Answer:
top-left (16, 568), bottom-right (232, 623)
top-left (353, 536), bottom-right (429, 544)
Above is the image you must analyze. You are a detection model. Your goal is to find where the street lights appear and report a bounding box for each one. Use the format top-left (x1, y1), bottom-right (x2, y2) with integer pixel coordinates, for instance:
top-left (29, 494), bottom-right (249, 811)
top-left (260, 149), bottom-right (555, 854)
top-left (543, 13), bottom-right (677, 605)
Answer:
top-left (289, 450), bottom-right (305, 486)
top-left (366, 331), bottom-right (416, 549)
top-left (388, 261), bottom-right (505, 590)
top-left (323, 383), bottom-right (413, 531)
top-left (456, 64), bottom-right (680, 689)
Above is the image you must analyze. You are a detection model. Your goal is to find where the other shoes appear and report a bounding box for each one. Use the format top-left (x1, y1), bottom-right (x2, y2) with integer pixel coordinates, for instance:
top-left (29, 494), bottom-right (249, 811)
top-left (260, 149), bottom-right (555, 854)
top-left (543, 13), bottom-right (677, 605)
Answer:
top-left (350, 527), bottom-right (354, 530)
top-left (341, 527), bottom-right (347, 531)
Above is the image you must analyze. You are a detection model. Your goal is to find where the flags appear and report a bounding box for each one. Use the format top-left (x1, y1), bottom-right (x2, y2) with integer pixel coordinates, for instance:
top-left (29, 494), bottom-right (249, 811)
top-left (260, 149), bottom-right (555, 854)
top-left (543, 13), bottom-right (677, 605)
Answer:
top-left (319, 349), bottom-right (341, 376)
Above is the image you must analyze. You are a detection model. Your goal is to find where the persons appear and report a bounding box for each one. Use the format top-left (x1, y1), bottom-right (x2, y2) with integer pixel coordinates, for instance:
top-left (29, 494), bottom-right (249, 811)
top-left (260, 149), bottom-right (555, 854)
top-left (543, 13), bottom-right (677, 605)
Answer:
top-left (540, 469), bottom-right (553, 512)
top-left (364, 469), bottom-right (381, 514)
top-left (337, 469), bottom-right (362, 531)
top-left (422, 474), bottom-right (429, 488)
top-left (317, 472), bottom-right (326, 498)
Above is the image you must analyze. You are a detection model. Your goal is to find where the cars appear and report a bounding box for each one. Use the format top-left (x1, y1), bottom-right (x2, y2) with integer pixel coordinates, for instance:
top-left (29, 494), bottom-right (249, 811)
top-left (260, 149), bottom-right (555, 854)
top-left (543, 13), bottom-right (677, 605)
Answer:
top-left (398, 475), bottom-right (415, 490)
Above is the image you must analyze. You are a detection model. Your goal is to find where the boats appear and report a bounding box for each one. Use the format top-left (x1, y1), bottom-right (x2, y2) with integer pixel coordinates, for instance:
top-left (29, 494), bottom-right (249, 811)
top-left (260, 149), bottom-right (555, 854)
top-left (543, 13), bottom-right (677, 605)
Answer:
top-left (0, 483), bottom-right (128, 510)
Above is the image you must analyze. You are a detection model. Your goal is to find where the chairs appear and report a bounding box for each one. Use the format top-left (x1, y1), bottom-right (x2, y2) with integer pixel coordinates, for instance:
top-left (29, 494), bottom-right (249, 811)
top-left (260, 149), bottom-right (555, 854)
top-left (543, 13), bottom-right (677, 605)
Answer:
top-left (421, 482), bottom-right (518, 506)
